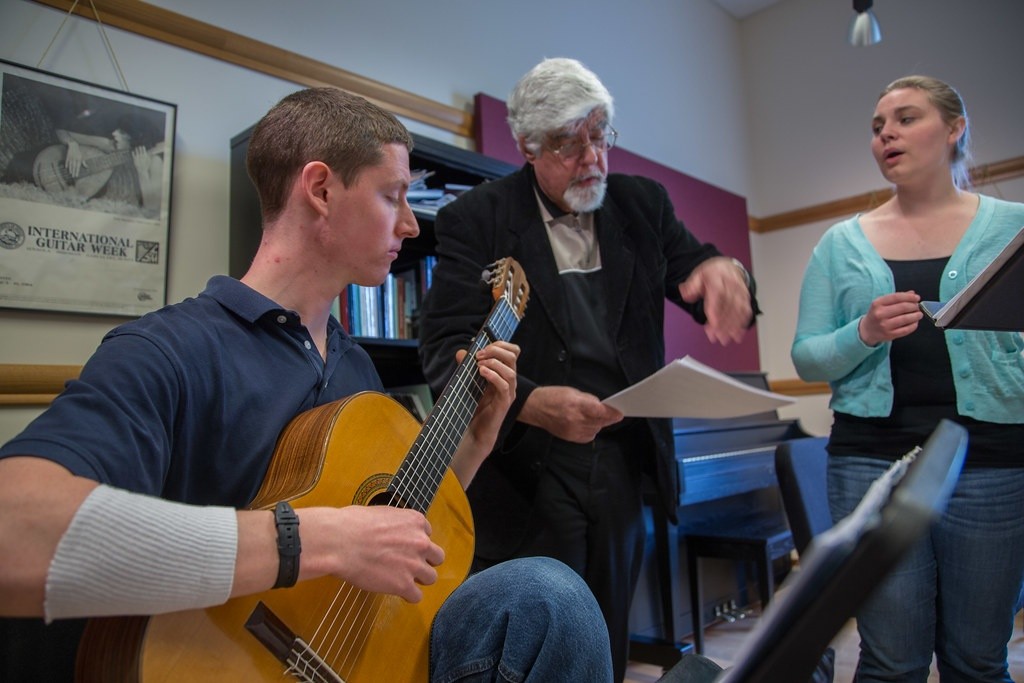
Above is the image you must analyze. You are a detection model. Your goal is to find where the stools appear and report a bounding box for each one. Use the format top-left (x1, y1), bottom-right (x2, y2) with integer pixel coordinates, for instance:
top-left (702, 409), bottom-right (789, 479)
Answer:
top-left (683, 484), bottom-right (796, 655)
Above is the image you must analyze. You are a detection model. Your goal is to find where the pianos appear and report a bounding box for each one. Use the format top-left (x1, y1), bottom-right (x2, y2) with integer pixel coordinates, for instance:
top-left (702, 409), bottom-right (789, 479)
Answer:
top-left (624, 372), bottom-right (816, 667)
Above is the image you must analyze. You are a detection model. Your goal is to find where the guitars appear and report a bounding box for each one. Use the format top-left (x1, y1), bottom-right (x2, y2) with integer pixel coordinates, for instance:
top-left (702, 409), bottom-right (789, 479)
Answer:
top-left (72, 257), bottom-right (531, 683)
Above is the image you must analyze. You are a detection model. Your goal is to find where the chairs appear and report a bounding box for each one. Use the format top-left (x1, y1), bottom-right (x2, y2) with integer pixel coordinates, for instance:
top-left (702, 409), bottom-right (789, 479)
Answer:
top-left (775, 436), bottom-right (841, 557)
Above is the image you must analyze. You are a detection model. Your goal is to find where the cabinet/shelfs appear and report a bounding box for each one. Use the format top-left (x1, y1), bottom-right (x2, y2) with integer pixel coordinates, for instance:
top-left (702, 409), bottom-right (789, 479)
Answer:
top-left (229, 122), bottom-right (523, 346)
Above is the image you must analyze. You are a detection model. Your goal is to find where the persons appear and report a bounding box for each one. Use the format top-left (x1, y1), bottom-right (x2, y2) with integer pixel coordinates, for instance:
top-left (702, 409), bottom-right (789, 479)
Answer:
top-left (792, 75), bottom-right (1024, 683)
top-left (1, 86), bottom-right (615, 682)
top-left (418, 58), bottom-right (761, 683)
top-left (6, 114), bottom-right (164, 210)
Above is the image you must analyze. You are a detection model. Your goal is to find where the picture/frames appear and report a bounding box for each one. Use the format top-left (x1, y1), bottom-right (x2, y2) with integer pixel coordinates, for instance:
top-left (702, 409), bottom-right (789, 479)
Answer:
top-left (0, 57), bottom-right (178, 318)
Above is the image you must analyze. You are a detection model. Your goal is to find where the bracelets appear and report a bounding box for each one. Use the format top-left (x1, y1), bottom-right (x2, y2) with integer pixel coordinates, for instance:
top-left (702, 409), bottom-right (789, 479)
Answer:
top-left (271, 503), bottom-right (300, 588)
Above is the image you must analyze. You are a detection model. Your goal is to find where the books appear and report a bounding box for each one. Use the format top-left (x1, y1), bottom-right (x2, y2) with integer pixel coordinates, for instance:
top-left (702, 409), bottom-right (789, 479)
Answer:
top-left (327, 256), bottom-right (434, 339)
top-left (407, 168), bottom-right (444, 209)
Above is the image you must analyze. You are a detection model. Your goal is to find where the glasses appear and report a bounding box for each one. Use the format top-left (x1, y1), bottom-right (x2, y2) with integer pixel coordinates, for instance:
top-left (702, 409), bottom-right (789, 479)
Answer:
top-left (543, 123), bottom-right (618, 161)
